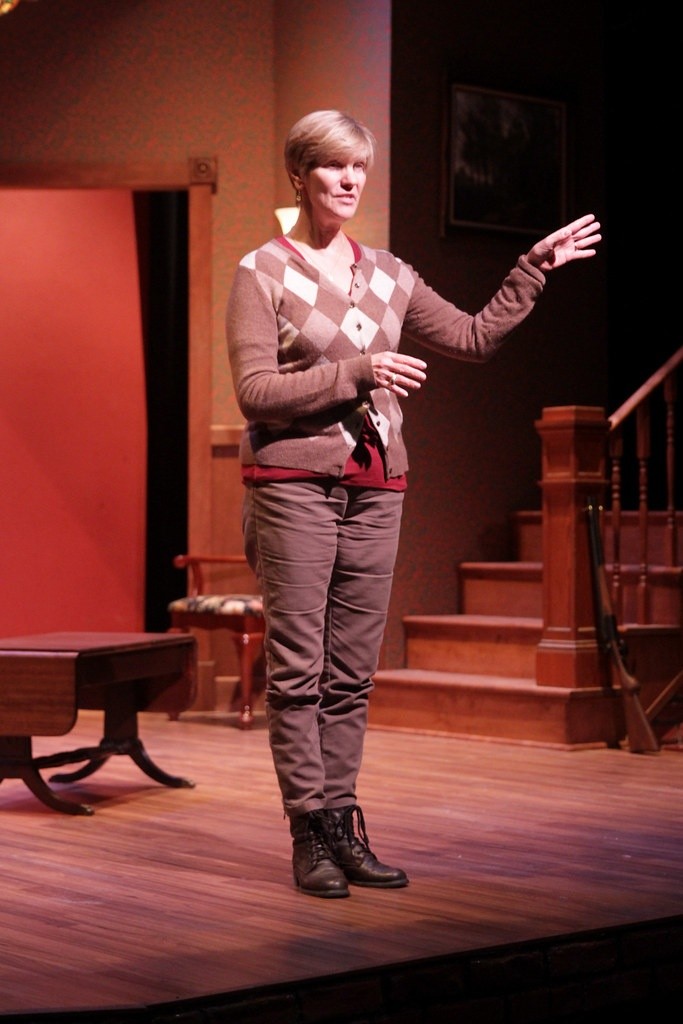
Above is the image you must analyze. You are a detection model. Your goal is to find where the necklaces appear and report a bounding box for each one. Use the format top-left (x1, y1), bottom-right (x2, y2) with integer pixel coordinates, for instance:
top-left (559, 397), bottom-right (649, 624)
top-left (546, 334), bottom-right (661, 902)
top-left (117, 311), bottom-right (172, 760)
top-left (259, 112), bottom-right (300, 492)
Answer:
top-left (288, 224), bottom-right (346, 284)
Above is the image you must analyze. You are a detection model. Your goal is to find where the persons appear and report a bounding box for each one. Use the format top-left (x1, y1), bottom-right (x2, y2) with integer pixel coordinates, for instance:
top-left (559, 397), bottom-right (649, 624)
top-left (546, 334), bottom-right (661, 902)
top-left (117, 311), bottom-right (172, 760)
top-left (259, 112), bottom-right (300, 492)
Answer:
top-left (226, 108), bottom-right (601, 899)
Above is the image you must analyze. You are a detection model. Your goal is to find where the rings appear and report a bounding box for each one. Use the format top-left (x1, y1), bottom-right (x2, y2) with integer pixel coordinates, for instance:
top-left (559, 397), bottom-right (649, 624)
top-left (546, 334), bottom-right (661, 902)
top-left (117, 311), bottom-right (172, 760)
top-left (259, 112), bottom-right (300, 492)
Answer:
top-left (390, 374), bottom-right (396, 385)
top-left (574, 242), bottom-right (578, 251)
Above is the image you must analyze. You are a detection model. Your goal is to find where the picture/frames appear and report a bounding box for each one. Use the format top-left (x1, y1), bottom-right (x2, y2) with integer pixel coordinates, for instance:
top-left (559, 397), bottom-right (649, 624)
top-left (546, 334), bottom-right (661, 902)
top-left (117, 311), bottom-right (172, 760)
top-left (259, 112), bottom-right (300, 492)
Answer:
top-left (441, 62), bottom-right (577, 242)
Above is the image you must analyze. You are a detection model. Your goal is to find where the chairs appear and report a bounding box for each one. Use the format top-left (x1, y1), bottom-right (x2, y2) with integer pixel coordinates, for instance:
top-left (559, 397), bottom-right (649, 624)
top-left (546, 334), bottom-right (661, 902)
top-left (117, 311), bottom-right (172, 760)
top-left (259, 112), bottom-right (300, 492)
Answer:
top-left (167, 555), bottom-right (269, 728)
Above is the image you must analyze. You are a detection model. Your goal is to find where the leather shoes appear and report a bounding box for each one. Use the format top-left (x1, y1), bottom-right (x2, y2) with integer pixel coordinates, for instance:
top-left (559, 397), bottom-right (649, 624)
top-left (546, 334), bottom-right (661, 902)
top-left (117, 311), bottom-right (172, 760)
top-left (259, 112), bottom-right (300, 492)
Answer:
top-left (289, 808), bottom-right (349, 897)
top-left (321, 805), bottom-right (410, 890)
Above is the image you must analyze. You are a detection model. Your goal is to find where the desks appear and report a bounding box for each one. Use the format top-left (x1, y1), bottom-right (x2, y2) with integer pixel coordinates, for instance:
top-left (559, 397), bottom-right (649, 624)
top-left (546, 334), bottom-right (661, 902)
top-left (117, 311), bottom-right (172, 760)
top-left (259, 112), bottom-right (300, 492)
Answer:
top-left (0, 630), bottom-right (196, 814)
